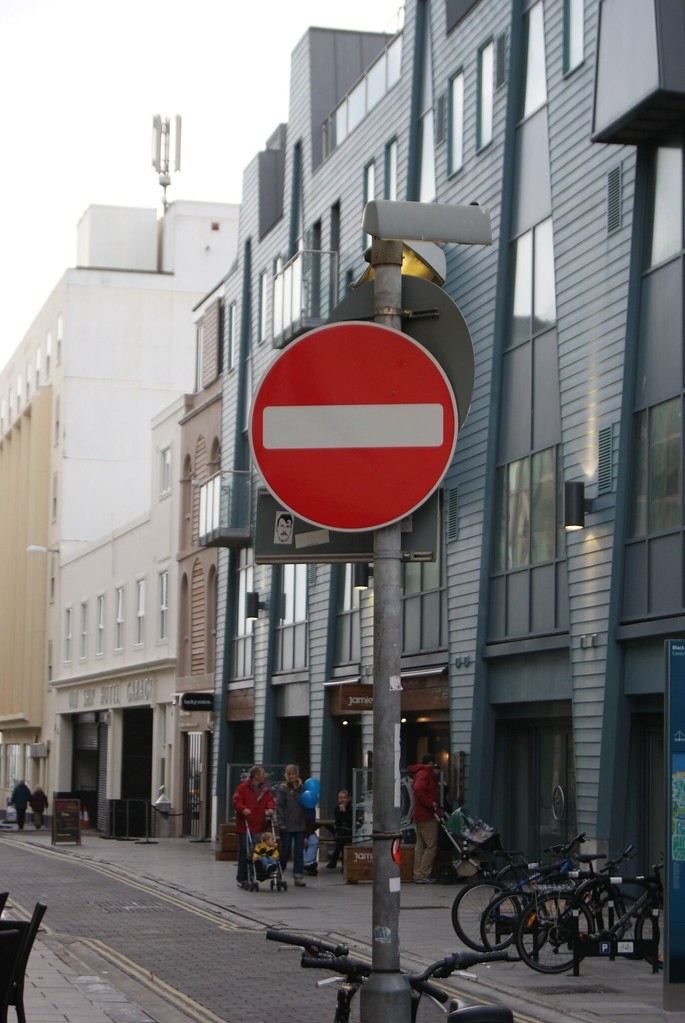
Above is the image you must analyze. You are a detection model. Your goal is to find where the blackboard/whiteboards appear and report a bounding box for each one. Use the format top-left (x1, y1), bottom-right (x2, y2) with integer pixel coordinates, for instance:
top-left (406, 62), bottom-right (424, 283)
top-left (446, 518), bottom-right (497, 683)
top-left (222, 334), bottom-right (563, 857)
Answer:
top-left (52, 798), bottom-right (81, 842)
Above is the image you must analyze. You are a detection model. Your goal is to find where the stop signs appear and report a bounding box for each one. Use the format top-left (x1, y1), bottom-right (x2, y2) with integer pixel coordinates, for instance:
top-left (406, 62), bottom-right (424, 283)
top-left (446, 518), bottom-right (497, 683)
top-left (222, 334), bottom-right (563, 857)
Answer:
top-left (248, 320), bottom-right (459, 534)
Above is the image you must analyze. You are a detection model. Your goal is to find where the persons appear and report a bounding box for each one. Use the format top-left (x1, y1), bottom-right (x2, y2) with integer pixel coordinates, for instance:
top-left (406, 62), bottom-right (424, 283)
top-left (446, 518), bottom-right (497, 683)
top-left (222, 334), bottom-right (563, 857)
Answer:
top-left (276, 764), bottom-right (316, 887)
top-left (407, 754), bottom-right (441, 884)
top-left (232, 765), bottom-right (278, 888)
top-left (10, 780), bottom-right (31, 829)
top-left (326, 790), bottom-right (357, 868)
top-left (252, 832), bottom-right (280, 872)
top-left (29, 785), bottom-right (48, 829)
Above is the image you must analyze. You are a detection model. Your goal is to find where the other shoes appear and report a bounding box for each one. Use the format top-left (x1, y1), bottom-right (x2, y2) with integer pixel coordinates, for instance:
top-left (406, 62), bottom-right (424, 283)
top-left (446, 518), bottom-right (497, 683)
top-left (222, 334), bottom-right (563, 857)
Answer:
top-left (238, 880), bottom-right (248, 889)
top-left (327, 863), bottom-right (337, 868)
top-left (266, 864), bottom-right (276, 875)
top-left (413, 875), bottom-right (438, 884)
top-left (295, 879), bottom-right (306, 887)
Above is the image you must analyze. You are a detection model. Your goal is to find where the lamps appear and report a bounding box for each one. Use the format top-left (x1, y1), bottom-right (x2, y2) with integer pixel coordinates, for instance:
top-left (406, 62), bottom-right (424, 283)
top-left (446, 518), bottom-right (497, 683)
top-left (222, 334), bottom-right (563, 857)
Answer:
top-left (27, 545), bottom-right (58, 553)
top-left (354, 561), bottom-right (374, 591)
top-left (565, 479), bottom-right (592, 530)
top-left (246, 591), bottom-right (263, 620)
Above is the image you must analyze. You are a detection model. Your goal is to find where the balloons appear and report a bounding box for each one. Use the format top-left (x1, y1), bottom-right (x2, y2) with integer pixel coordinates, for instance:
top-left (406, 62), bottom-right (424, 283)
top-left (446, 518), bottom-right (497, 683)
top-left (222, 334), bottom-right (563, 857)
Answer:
top-left (304, 778), bottom-right (321, 794)
top-left (300, 790), bottom-right (318, 808)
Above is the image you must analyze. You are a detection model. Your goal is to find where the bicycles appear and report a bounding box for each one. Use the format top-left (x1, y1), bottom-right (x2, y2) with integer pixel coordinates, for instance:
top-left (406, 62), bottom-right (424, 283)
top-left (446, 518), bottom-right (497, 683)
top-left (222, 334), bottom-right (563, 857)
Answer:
top-left (266, 929), bottom-right (513, 1023)
top-left (451, 832), bottom-right (665, 974)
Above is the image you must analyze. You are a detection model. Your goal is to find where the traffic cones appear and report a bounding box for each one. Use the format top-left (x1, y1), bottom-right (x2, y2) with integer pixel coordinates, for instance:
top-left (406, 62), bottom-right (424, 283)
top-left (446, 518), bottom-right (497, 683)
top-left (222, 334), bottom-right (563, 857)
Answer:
top-left (80, 805), bottom-right (89, 829)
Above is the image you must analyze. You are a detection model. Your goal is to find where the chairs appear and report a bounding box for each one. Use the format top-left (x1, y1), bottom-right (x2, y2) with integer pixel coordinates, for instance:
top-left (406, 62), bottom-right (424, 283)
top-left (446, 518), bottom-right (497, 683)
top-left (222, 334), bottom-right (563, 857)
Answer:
top-left (251, 831), bottom-right (280, 869)
top-left (0, 891), bottom-right (48, 1023)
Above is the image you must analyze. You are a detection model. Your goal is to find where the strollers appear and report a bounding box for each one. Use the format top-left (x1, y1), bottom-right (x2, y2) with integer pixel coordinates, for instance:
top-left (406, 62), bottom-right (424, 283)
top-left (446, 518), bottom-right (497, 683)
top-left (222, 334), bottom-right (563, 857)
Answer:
top-left (432, 805), bottom-right (504, 886)
top-left (242, 813), bottom-right (288, 893)
